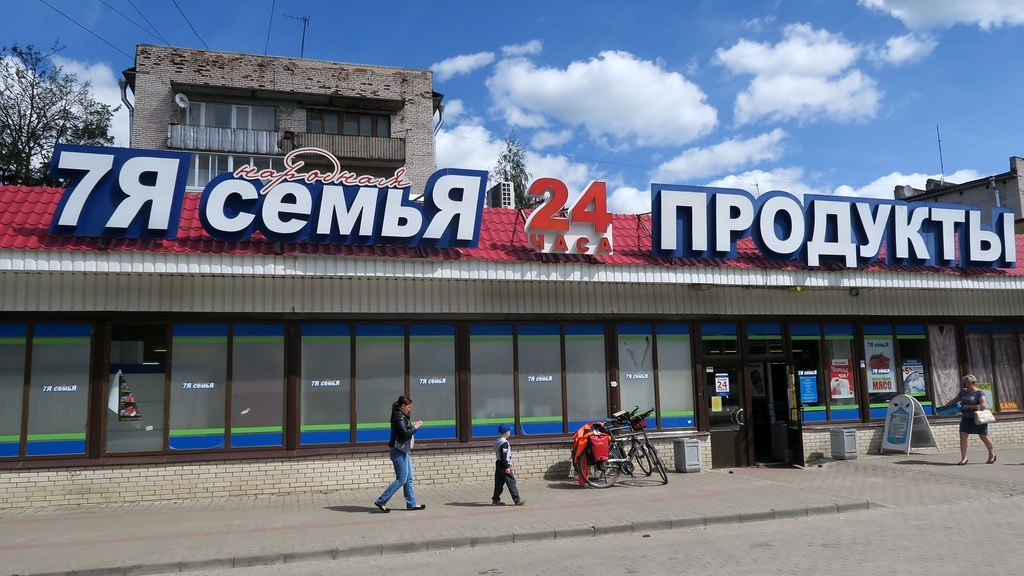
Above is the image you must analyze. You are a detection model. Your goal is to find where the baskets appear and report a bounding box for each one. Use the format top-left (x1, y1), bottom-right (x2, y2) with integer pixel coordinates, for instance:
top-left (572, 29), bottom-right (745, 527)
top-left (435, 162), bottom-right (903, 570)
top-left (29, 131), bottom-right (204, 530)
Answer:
top-left (613, 409), bottom-right (630, 425)
top-left (628, 413), bottom-right (647, 431)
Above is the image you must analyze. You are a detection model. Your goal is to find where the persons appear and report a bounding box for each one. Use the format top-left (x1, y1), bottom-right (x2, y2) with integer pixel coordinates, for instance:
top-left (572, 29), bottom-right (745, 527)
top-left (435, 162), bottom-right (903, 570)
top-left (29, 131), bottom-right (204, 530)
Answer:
top-left (933, 375), bottom-right (997, 465)
top-left (491, 423), bottom-right (524, 506)
top-left (375, 395), bottom-right (426, 513)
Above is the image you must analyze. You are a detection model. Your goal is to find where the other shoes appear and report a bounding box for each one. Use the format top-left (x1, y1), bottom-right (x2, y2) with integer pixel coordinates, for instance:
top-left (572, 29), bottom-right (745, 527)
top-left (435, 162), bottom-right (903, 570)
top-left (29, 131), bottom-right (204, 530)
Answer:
top-left (411, 504), bottom-right (425, 510)
top-left (492, 500), bottom-right (505, 506)
top-left (515, 500), bottom-right (527, 506)
top-left (375, 502), bottom-right (390, 513)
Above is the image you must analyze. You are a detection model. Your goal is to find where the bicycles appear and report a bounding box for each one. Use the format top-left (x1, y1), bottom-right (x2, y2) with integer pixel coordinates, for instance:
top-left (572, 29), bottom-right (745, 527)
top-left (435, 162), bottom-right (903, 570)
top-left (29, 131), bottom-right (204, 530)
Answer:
top-left (567, 405), bottom-right (668, 489)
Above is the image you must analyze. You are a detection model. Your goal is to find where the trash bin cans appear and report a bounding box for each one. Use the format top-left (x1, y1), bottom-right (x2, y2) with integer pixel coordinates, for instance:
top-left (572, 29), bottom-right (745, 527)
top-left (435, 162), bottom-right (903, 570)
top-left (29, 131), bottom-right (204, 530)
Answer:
top-left (831, 426), bottom-right (858, 460)
top-left (674, 438), bottom-right (701, 474)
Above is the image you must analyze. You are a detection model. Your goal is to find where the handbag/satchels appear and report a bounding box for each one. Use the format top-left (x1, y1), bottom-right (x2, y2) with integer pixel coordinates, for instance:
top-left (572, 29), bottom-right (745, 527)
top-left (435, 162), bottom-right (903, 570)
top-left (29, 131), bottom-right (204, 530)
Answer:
top-left (410, 434), bottom-right (415, 449)
top-left (974, 409), bottom-right (996, 425)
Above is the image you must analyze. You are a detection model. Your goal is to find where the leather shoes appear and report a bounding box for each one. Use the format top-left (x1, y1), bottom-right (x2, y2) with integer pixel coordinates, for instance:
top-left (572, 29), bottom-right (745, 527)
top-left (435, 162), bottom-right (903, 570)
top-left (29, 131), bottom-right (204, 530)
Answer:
top-left (985, 454), bottom-right (996, 464)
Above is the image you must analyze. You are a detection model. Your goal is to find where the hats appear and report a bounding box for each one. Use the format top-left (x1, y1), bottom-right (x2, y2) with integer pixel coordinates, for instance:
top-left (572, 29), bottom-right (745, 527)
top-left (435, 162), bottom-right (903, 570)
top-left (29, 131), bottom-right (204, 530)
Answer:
top-left (499, 423), bottom-right (514, 434)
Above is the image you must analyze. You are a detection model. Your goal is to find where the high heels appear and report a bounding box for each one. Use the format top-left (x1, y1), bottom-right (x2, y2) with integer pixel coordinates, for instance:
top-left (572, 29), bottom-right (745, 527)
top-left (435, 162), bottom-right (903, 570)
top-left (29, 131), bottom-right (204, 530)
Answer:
top-left (958, 459), bottom-right (968, 465)
